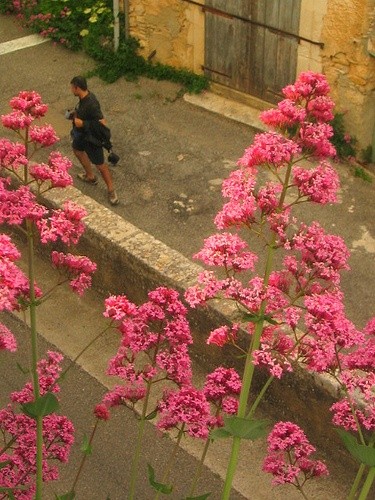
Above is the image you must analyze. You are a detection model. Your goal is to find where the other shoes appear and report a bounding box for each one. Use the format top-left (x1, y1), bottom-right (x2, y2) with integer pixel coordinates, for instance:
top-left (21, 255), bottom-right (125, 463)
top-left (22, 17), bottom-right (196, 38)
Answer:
top-left (108, 188), bottom-right (120, 206)
top-left (76, 173), bottom-right (99, 185)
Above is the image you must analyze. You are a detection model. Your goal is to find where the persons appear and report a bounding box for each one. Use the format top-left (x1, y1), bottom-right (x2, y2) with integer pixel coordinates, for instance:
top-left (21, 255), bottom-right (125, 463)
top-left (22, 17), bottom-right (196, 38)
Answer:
top-left (70, 76), bottom-right (119, 206)
